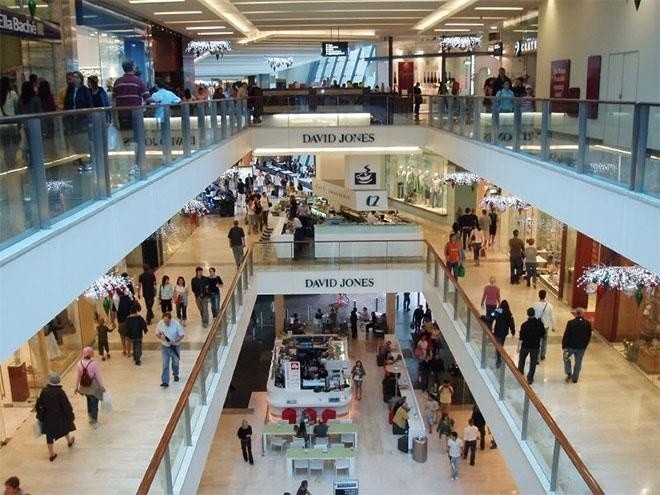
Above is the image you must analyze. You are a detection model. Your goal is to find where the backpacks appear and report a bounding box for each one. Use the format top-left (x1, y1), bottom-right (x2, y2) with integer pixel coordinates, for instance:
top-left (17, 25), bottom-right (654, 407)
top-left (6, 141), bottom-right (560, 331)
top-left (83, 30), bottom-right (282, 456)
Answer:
top-left (79, 360), bottom-right (94, 387)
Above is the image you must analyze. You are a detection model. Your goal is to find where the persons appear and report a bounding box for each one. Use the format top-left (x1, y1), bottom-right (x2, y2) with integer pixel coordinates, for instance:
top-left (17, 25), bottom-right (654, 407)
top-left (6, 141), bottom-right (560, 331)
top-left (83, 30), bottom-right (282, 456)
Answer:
top-left (34, 263), bottom-right (223, 463)
top-left (201, 159), bottom-right (395, 268)
top-left (395, 166), bottom-right (443, 208)
top-left (444, 207), bottom-right (592, 384)
top-left (0, 60), bottom-right (265, 212)
top-left (236, 293), bottom-right (496, 465)
top-left (447, 432), bottom-right (465, 481)
top-left (297, 480), bottom-right (310, 493)
top-left (4, 478), bottom-right (28, 494)
top-left (414, 67), bottom-right (535, 140)
top-left (288, 79), bottom-right (379, 111)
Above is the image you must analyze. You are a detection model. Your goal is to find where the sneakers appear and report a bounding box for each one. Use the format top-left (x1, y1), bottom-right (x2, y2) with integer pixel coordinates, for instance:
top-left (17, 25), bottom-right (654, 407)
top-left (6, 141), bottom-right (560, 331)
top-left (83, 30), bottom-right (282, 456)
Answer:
top-left (49, 418), bottom-right (99, 462)
top-left (527, 356), bottom-right (580, 384)
top-left (464, 242), bottom-right (494, 266)
top-left (511, 281), bottom-right (537, 289)
top-left (101, 318), bottom-right (187, 388)
top-left (450, 448), bottom-right (485, 482)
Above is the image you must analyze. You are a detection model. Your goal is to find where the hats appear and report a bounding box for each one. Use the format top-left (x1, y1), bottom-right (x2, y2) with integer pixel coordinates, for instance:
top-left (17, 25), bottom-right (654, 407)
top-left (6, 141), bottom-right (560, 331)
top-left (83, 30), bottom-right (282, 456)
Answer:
top-left (83, 347), bottom-right (94, 358)
top-left (48, 373), bottom-right (63, 386)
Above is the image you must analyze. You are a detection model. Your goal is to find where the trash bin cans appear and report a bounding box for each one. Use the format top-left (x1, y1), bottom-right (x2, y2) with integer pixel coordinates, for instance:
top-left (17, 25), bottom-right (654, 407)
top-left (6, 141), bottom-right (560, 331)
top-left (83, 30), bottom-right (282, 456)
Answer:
top-left (413, 436), bottom-right (428, 463)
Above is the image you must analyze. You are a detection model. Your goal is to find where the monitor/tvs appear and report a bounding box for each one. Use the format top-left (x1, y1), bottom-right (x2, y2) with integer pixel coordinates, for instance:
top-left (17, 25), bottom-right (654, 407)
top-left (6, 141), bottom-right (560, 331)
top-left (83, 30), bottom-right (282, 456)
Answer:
top-left (322, 42), bottom-right (348, 56)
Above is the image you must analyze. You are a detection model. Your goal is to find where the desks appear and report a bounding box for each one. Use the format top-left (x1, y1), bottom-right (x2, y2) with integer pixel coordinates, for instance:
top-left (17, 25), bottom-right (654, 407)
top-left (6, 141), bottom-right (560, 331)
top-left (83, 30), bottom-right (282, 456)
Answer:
top-left (285, 447), bottom-right (356, 480)
top-left (384, 333), bottom-right (426, 452)
top-left (262, 424), bottom-right (357, 453)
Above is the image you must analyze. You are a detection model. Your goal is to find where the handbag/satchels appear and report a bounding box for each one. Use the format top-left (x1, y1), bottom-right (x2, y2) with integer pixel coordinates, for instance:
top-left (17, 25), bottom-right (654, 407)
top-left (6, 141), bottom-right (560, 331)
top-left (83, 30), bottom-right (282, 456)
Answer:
top-left (458, 263), bottom-right (465, 277)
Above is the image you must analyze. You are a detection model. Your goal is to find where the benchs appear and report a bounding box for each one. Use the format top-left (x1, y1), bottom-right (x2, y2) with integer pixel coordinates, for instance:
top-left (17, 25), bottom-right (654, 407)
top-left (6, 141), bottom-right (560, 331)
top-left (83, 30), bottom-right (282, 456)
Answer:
top-left (373, 313), bottom-right (387, 338)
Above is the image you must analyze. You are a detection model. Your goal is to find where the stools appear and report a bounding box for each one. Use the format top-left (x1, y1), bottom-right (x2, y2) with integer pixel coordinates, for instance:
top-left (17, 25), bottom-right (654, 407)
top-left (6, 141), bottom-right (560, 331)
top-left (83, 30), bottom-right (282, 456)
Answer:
top-left (377, 354), bottom-right (384, 366)
top-left (393, 423), bottom-right (403, 434)
top-left (389, 411), bottom-right (394, 424)
top-left (398, 436), bottom-right (407, 452)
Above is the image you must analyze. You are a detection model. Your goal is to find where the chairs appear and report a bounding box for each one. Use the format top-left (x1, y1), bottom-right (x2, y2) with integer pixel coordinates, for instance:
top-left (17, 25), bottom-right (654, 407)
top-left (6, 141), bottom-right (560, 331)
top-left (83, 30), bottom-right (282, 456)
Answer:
top-left (294, 458), bottom-right (350, 481)
top-left (269, 430), bottom-right (355, 456)
top-left (275, 417), bottom-right (352, 425)
